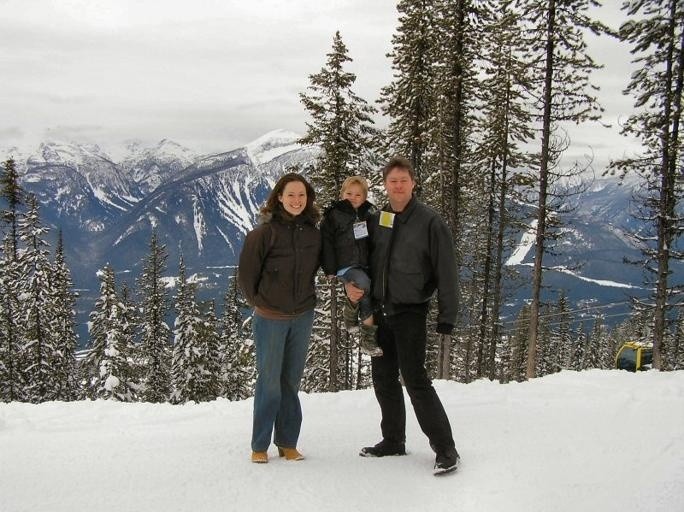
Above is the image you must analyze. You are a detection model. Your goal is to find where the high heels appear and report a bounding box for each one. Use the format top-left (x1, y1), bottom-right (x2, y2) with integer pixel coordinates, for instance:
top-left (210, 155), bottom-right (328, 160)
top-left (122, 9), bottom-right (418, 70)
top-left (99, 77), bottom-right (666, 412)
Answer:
top-left (278, 446), bottom-right (305, 460)
top-left (252, 451), bottom-right (268, 462)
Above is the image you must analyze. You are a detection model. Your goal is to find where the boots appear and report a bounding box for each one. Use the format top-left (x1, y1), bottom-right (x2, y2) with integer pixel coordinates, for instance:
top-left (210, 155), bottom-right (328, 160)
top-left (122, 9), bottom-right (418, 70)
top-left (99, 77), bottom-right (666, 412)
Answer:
top-left (359, 322), bottom-right (383, 357)
top-left (344, 297), bottom-right (361, 333)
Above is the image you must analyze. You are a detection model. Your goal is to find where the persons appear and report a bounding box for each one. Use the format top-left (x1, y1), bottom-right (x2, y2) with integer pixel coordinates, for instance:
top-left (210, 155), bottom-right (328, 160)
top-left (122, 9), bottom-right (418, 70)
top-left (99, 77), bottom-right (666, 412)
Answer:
top-left (233, 171), bottom-right (326, 464)
top-left (318, 174), bottom-right (385, 358)
top-left (343, 154), bottom-right (461, 476)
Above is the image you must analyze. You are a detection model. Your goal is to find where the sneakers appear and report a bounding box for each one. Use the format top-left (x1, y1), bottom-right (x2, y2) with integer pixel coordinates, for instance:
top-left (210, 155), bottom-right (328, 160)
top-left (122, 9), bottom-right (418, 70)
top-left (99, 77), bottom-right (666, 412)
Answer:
top-left (359, 440), bottom-right (406, 457)
top-left (434, 448), bottom-right (460, 476)
